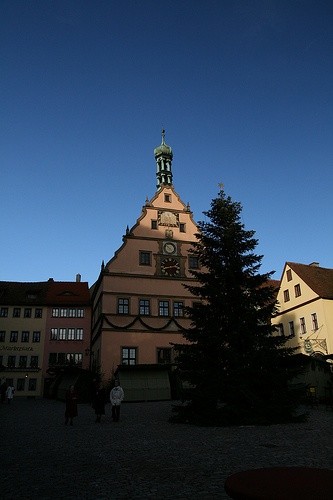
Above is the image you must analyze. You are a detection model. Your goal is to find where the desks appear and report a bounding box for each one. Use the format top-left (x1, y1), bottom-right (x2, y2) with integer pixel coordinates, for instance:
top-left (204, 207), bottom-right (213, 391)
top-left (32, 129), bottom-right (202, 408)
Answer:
top-left (223, 466), bottom-right (333, 500)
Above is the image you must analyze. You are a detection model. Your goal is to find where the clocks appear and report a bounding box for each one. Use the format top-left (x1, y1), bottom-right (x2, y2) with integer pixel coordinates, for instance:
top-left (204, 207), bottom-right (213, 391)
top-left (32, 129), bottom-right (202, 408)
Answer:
top-left (164, 241), bottom-right (177, 256)
top-left (160, 257), bottom-right (181, 277)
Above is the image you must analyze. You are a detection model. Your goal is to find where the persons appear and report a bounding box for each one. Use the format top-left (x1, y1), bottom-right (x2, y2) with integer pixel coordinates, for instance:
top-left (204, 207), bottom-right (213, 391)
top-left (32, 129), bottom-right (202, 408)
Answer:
top-left (66, 384), bottom-right (78, 429)
top-left (6, 383), bottom-right (14, 404)
top-left (110, 380), bottom-right (124, 422)
top-left (92, 382), bottom-right (106, 424)
